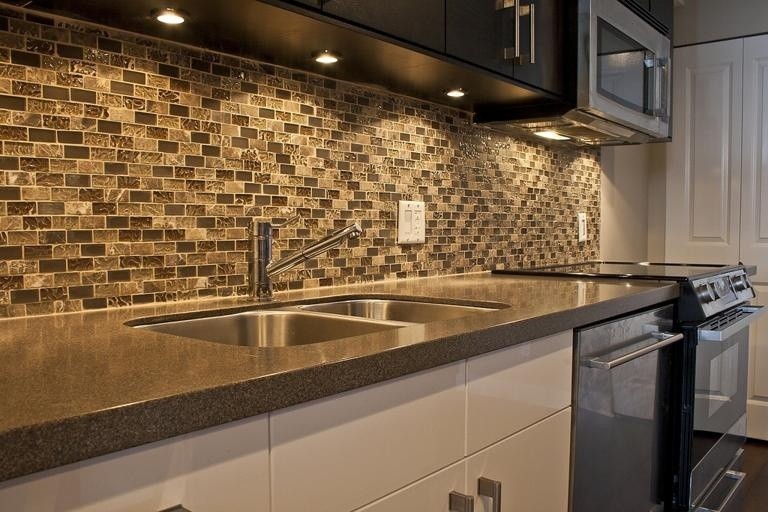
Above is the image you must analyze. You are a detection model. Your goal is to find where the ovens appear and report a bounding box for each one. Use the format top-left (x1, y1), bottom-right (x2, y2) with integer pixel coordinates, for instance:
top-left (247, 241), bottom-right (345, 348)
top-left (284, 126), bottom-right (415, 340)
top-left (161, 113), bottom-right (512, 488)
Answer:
top-left (668, 304), bottom-right (767, 512)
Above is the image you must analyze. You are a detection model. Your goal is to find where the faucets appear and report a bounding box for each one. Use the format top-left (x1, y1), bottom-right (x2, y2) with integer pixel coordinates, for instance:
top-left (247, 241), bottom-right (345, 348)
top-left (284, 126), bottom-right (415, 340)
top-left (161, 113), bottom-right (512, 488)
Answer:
top-left (247, 215), bottom-right (364, 298)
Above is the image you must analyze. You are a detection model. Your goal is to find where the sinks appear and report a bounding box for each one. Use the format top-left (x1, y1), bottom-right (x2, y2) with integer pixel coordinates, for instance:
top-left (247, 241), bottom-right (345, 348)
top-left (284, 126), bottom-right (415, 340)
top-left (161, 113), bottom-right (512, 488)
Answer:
top-left (129, 310), bottom-right (409, 348)
top-left (296, 298), bottom-right (505, 325)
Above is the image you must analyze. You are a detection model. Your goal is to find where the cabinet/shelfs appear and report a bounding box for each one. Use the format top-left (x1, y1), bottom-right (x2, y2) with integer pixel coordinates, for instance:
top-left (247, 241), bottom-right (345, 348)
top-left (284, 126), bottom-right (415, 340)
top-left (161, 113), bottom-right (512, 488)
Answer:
top-left (663, 34), bottom-right (768, 441)
top-left (0, 0), bottom-right (579, 115)
top-left (1, 411), bottom-right (270, 511)
top-left (268, 283), bottom-right (573, 512)
top-left (618, 0), bottom-right (673, 39)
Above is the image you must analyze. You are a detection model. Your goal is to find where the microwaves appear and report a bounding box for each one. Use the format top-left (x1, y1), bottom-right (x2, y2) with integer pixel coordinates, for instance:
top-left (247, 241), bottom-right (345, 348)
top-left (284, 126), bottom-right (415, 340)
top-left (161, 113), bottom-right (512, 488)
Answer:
top-left (465, 0), bottom-right (675, 152)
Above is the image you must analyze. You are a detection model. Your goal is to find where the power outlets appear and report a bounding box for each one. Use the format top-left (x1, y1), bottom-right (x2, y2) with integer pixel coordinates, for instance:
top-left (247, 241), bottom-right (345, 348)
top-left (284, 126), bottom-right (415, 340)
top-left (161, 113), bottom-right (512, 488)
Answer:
top-left (398, 200), bottom-right (426, 244)
